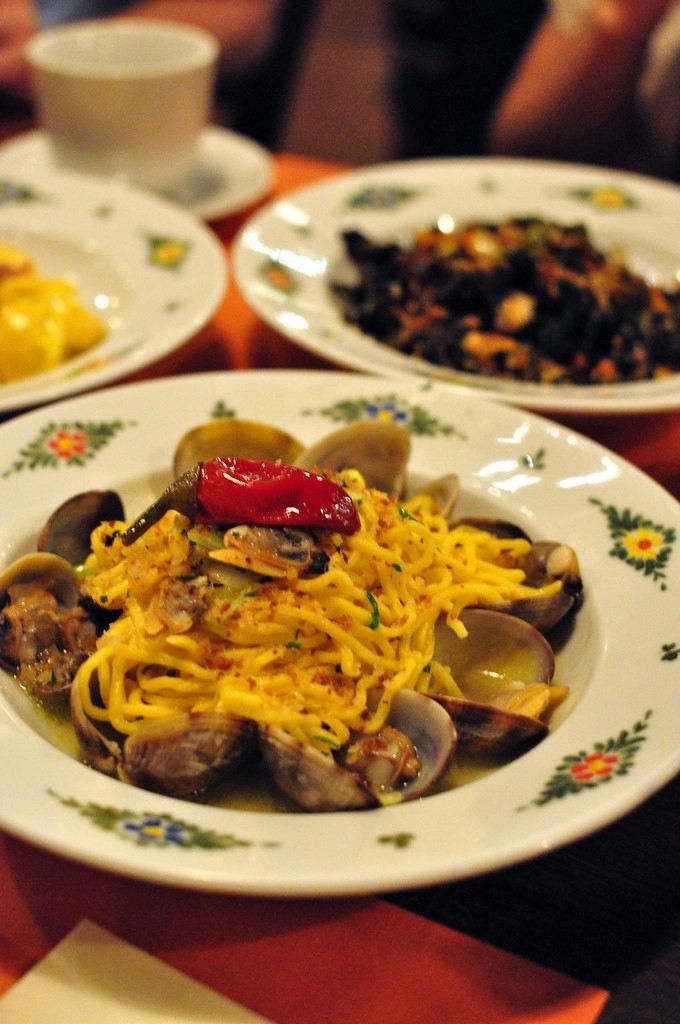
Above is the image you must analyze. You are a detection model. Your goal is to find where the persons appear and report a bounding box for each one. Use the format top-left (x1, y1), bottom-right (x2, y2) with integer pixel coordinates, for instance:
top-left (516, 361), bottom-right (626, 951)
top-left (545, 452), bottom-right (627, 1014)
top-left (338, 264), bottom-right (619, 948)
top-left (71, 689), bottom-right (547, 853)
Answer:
top-left (494, 0), bottom-right (680, 191)
top-left (2, 0), bottom-right (551, 164)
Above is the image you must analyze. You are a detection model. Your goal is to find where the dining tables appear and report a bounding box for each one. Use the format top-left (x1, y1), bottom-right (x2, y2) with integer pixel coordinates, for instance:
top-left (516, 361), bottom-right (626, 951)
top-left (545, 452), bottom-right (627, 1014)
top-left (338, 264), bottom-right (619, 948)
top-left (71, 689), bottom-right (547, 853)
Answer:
top-left (0, 100), bottom-right (680, 1024)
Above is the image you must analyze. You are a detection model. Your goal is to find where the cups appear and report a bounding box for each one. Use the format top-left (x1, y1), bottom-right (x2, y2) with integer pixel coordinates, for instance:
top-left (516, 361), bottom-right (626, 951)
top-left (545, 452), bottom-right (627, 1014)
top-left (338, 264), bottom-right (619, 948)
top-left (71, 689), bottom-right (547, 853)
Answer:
top-left (21, 21), bottom-right (218, 199)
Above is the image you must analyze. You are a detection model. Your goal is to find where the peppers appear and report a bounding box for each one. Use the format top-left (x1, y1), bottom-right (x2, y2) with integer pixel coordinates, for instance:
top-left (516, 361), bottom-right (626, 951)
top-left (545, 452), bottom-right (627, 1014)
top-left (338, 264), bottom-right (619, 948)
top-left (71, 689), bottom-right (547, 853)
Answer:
top-left (120, 455), bottom-right (361, 544)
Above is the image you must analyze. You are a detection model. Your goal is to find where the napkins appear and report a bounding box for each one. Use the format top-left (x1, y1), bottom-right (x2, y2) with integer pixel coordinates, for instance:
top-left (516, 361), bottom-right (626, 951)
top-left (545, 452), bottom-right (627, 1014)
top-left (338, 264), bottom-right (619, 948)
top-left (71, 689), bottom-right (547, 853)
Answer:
top-left (0, 920), bottom-right (274, 1023)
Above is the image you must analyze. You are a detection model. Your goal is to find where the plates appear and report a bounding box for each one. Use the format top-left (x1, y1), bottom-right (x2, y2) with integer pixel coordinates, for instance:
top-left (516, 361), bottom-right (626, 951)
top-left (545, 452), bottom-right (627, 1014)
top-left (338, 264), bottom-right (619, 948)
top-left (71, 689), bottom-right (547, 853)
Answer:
top-left (0, 166), bottom-right (230, 413)
top-left (0, 120), bottom-right (278, 225)
top-left (0, 370), bottom-right (680, 898)
top-left (230, 155), bottom-right (680, 418)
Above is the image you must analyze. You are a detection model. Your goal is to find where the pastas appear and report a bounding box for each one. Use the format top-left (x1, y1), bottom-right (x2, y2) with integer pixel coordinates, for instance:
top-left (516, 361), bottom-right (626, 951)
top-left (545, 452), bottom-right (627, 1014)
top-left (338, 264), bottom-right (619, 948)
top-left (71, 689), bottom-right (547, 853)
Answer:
top-left (74, 473), bottom-right (562, 753)
